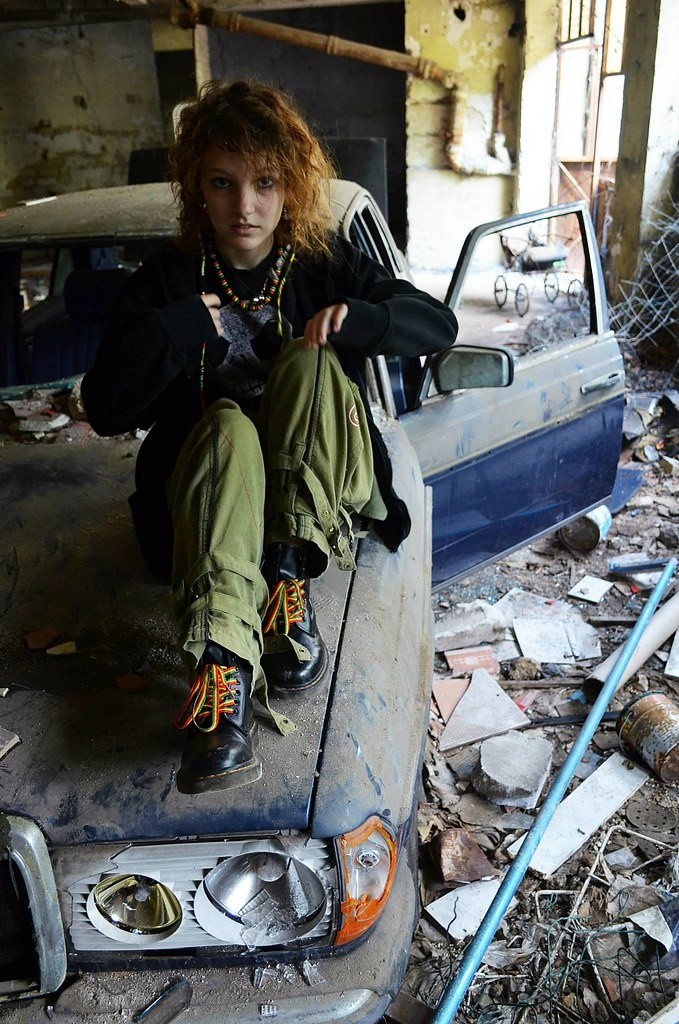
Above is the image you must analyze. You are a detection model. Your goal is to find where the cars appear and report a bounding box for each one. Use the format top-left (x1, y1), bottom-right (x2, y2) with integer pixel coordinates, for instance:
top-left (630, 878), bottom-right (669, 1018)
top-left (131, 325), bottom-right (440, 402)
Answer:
top-left (0, 179), bottom-right (626, 1024)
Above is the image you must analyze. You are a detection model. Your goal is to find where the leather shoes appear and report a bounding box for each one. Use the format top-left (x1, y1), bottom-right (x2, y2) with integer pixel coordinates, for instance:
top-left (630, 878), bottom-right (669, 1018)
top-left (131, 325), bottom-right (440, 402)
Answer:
top-left (259, 541), bottom-right (330, 696)
top-left (180, 644), bottom-right (265, 793)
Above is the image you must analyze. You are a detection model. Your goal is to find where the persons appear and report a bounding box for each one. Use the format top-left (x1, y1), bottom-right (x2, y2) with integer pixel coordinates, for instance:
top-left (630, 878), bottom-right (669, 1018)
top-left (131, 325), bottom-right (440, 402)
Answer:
top-left (78, 77), bottom-right (458, 795)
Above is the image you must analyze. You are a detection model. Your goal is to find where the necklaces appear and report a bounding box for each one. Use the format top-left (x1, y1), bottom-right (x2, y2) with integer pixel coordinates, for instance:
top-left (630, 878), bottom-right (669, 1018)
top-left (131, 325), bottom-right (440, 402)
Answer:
top-left (189, 220), bottom-right (299, 418)
